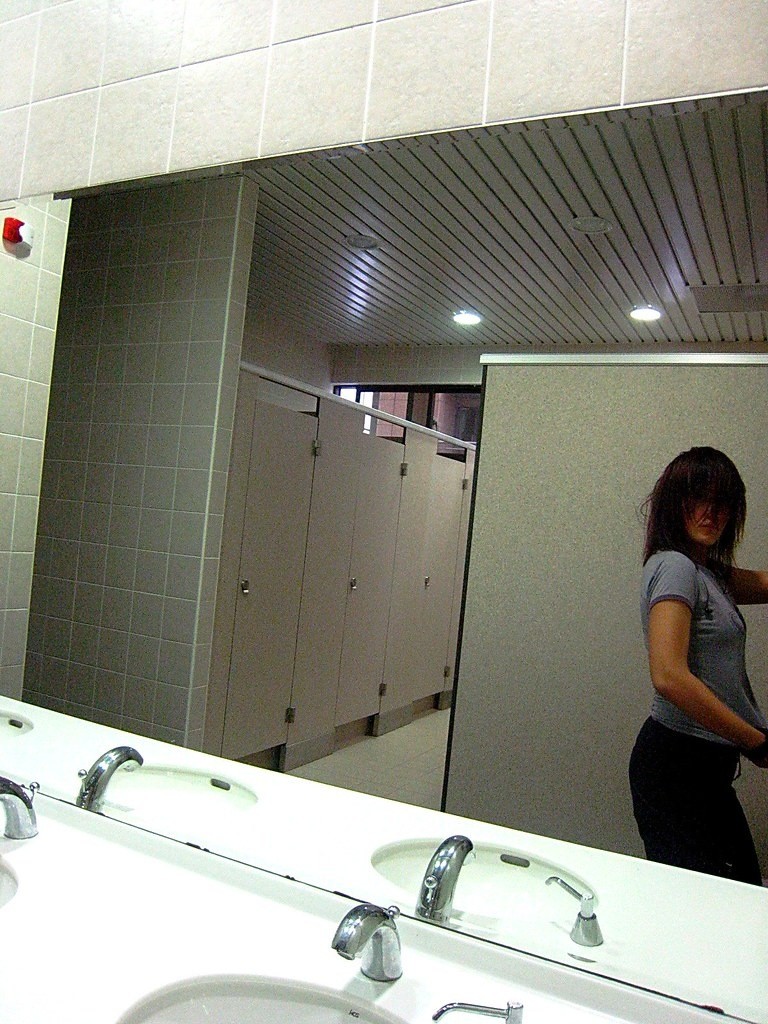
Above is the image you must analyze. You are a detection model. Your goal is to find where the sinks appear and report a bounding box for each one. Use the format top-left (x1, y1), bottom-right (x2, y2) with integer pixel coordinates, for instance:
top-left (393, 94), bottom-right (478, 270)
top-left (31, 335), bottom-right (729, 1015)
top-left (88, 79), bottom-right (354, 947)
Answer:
top-left (0, 855), bottom-right (15, 911)
top-left (370, 837), bottom-right (599, 920)
top-left (115, 972), bottom-right (409, 1023)
top-left (0, 708), bottom-right (36, 741)
top-left (100, 764), bottom-right (259, 821)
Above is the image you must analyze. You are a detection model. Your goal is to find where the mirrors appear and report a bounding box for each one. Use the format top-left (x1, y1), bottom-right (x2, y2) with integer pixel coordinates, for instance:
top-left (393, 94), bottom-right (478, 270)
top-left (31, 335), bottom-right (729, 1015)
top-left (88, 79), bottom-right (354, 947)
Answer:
top-left (0, 88), bottom-right (768, 889)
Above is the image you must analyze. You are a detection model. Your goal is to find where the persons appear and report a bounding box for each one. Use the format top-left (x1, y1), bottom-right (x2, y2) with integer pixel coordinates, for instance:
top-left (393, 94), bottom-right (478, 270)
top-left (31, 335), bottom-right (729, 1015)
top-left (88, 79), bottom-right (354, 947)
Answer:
top-left (626, 445), bottom-right (767, 886)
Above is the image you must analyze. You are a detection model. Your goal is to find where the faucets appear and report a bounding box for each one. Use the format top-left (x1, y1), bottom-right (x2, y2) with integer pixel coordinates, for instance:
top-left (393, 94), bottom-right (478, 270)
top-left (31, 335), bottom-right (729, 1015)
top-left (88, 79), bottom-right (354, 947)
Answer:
top-left (545, 876), bottom-right (604, 947)
top-left (75, 744), bottom-right (144, 812)
top-left (416, 834), bottom-right (474, 922)
top-left (431, 1000), bottom-right (524, 1024)
top-left (0, 775), bottom-right (40, 840)
top-left (331, 903), bottom-right (402, 981)
